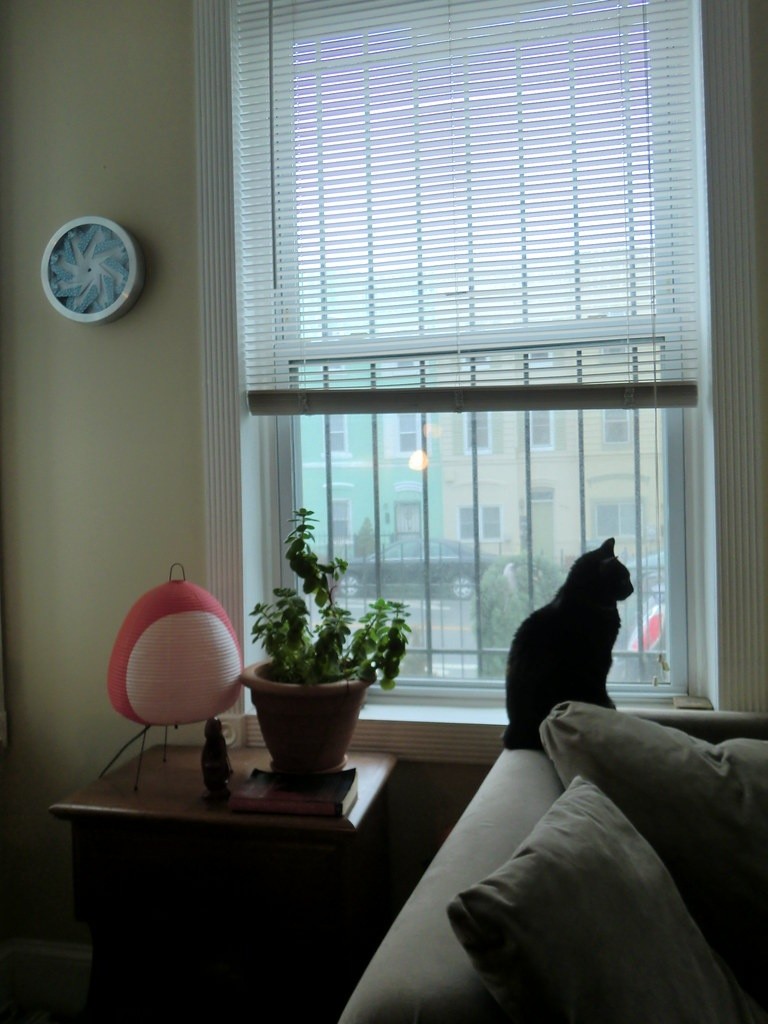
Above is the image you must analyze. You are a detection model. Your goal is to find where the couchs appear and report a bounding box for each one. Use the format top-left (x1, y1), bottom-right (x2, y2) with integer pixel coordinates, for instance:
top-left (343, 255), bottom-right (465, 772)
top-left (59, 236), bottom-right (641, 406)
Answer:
top-left (339, 707), bottom-right (767, 1024)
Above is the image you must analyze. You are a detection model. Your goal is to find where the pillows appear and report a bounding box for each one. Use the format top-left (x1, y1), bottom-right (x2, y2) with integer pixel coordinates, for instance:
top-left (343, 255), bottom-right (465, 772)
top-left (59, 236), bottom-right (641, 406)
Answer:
top-left (445, 703), bottom-right (768, 1024)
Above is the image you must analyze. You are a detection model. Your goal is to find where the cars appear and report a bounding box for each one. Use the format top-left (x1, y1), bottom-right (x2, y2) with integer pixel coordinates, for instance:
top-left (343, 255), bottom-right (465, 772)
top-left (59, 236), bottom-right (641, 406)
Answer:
top-left (624, 552), bottom-right (667, 656)
top-left (332, 538), bottom-right (496, 601)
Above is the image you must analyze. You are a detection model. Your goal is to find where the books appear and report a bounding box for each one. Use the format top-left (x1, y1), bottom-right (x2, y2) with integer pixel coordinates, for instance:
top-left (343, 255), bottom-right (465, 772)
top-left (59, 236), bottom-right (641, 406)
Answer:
top-left (228, 768), bottom-right (357, 817)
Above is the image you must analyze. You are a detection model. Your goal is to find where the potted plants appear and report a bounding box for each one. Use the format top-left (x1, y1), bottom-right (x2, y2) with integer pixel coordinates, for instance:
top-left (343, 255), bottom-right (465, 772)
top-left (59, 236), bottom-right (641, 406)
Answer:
top-left (238, 508), bottom-right (412, 785)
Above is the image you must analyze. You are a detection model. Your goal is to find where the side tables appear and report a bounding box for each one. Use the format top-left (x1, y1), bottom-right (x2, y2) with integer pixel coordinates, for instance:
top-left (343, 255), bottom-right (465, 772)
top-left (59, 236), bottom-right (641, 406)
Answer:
top-left (48, 744), bottom-right (400, 1024)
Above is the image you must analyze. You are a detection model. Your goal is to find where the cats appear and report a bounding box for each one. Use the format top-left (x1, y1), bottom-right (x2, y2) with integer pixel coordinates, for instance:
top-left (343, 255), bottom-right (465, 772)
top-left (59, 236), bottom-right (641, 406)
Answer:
top-left (500, 537), bottom-right (635, 752)
